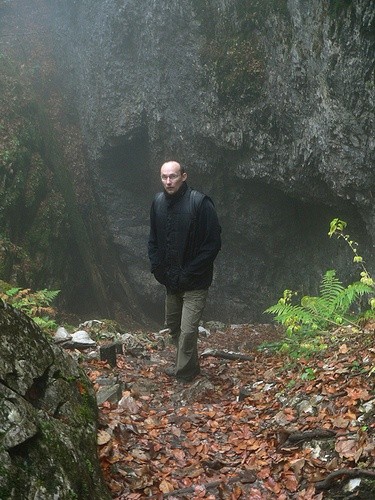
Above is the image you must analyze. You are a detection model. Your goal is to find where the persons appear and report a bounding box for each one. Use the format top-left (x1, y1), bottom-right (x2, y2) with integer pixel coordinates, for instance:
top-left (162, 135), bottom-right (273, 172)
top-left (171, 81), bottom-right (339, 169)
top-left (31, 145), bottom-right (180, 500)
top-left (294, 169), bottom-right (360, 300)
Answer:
top-left (147, 160), bottom-right (224, 383)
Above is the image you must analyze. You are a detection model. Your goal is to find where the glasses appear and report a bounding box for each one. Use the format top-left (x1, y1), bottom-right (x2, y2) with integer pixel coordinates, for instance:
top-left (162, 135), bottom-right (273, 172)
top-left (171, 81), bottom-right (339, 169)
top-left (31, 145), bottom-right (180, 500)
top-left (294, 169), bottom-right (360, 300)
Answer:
top-left (161, 175), bottom-right (179, 179)
